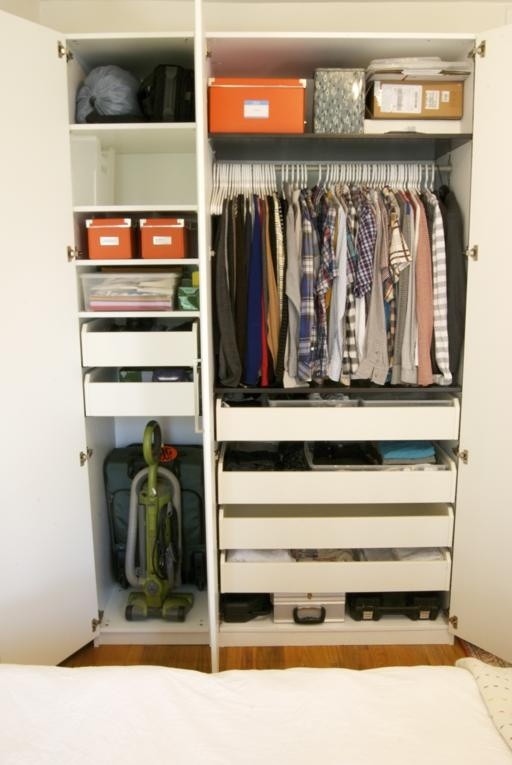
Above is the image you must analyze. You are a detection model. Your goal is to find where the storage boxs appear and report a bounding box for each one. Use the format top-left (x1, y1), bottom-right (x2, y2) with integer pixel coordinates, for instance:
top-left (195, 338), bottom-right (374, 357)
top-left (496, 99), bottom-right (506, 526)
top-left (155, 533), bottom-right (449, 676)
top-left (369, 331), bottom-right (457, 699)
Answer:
top-left (83, 217), bottom-right (132, 259)
top-left (206, 77), bottom-right (306, 132)
top-left (140, 218), bottom-right (186, 259)
top-left (311, 68), bottom-right (365, 136)
top-left (366, 81), bottom-right (464, 119)
top-left (79, 272), bottom-right (181, 311)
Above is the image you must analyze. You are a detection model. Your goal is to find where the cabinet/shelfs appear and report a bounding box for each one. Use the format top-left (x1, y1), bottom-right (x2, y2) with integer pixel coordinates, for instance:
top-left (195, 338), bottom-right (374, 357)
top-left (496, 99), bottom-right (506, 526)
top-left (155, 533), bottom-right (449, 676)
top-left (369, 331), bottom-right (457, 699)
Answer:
top-left (60, 31), bottom-right (480, 648)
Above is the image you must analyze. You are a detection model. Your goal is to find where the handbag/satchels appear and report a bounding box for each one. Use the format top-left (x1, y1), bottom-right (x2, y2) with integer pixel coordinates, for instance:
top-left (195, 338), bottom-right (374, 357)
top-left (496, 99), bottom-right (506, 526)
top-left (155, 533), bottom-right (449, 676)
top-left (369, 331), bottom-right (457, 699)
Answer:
top-left (75, 63), bottom-right (194, 123)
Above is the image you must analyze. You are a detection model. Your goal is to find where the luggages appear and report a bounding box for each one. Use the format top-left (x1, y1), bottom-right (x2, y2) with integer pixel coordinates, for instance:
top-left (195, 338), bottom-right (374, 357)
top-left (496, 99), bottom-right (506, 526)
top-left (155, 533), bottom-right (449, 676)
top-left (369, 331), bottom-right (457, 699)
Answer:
top-left (102, 420), bottom-right (206, 623)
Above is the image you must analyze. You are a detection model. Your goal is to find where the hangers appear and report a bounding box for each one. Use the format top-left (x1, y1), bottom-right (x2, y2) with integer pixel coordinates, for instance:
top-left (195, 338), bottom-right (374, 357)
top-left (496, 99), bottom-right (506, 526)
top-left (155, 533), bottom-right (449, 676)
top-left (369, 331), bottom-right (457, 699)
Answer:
top-left (210, 162), bottom-right (436, 216)
